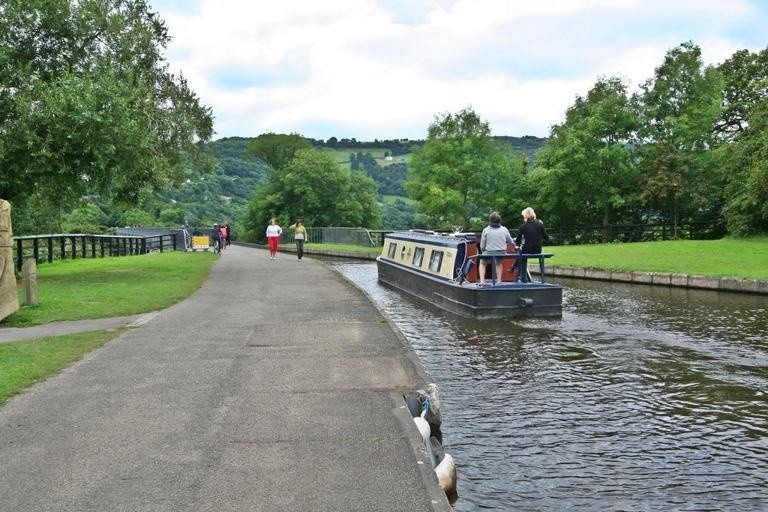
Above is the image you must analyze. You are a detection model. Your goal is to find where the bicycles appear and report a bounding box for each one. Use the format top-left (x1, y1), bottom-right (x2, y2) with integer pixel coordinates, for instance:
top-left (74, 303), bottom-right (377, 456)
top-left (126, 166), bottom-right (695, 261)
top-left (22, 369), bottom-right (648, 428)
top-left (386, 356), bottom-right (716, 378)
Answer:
top-left (212, 237), bottom-right (231, 254)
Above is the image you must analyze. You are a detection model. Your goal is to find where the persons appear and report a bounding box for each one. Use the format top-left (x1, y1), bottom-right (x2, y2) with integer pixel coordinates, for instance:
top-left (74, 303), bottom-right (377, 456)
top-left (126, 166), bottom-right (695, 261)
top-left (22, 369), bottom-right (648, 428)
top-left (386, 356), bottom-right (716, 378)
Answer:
top-left (266, 217), bottom-right (282, 260)
top-left (193, 223), bottom-right (230, 252)
top-left (289, 219), bottom-right (307, 261)
top-left (507, 207), bottom-right (548, 283)
top-left (479, 211), bottom-right (520, 284)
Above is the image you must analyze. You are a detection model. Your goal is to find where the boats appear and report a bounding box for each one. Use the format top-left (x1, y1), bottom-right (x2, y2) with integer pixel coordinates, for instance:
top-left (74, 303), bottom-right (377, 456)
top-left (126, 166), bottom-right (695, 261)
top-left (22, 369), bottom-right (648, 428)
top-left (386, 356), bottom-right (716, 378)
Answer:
top-left (373, 226), bottom-right (564, 321)
